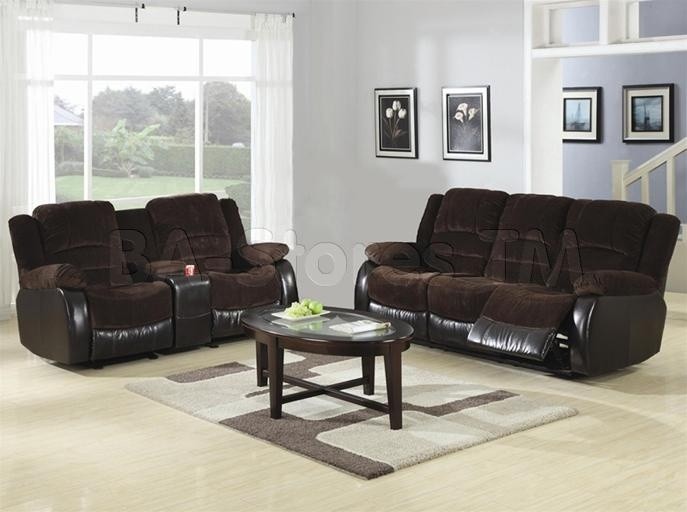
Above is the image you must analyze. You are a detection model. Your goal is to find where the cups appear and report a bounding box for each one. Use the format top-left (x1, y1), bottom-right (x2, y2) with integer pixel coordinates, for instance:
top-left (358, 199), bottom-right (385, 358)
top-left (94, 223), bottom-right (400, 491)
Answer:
top-left (185, 265), bottom-right (195, 277)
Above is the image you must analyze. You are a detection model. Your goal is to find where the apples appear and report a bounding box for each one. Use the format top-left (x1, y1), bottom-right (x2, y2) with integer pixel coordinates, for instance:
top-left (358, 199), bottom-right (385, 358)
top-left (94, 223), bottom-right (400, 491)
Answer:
top-left (309, 300), bottom-right (323, 314)
top-left (302, 299), bottom-right (311, 308)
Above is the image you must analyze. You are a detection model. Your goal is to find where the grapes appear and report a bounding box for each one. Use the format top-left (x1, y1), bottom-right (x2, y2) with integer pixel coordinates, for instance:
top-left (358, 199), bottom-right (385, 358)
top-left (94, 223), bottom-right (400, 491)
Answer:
top-left (285, 301), bottom-right (312, 318)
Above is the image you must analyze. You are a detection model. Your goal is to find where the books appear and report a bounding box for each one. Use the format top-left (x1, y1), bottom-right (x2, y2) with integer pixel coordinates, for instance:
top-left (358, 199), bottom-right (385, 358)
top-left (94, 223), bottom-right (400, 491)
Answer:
top-left (327, 319), bottom-right (392, 334)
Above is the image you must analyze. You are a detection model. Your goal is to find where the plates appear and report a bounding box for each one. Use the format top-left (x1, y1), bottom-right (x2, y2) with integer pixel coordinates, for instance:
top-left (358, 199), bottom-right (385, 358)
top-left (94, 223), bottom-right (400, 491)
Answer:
top-left (271, 309), bottom-right (331, 321)
top-left (271, 317), bottom-right (331, 329)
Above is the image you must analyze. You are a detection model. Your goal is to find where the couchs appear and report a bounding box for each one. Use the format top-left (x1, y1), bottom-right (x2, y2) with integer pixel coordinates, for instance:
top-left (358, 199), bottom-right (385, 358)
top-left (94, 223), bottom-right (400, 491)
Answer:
top-left (353, 189), bottom-right (681, 378)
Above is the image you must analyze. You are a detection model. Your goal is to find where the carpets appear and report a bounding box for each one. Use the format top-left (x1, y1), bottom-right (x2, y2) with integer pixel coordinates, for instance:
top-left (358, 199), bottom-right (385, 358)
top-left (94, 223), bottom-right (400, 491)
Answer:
top-left (125, 350), bottom-right (579, 481)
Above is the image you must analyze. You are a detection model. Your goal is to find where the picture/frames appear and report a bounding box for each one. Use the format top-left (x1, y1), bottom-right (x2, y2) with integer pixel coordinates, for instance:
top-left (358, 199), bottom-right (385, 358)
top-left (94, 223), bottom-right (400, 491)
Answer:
top-left (373, 85), bottom-right (491, 162)
top-left (562, 83), bottom-right (675, 144)
